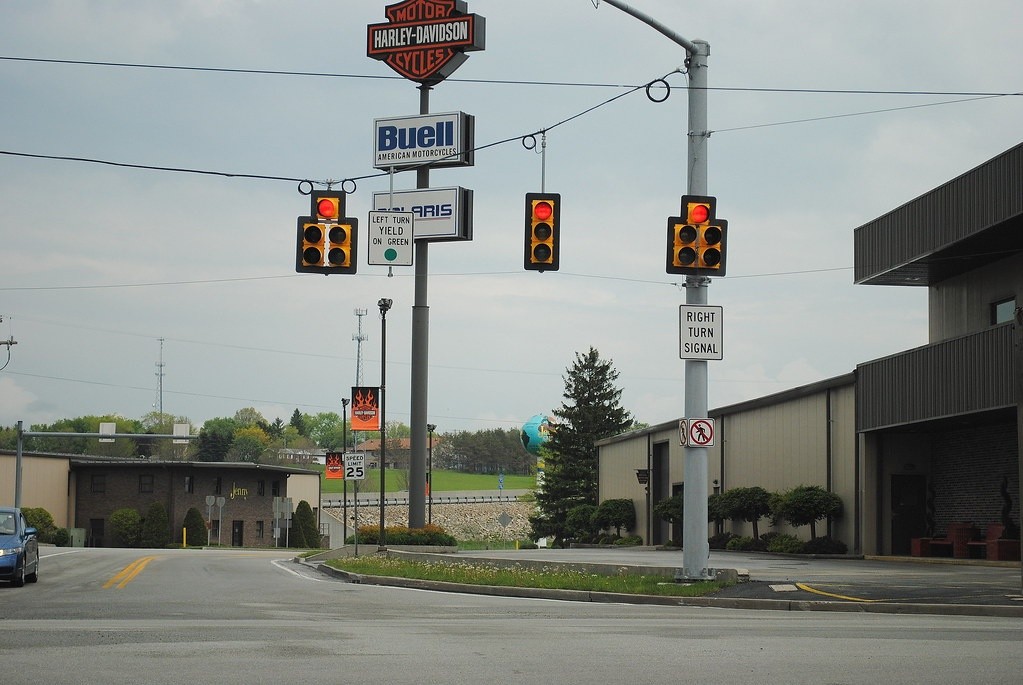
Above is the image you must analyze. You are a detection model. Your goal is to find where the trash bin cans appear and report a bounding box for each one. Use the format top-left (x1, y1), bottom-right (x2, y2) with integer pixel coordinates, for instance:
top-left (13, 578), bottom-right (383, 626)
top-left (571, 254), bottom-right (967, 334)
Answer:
top-left (953, 528), bottom-right (982, 559)
top-left (70, 528), bottom-right (86, 548)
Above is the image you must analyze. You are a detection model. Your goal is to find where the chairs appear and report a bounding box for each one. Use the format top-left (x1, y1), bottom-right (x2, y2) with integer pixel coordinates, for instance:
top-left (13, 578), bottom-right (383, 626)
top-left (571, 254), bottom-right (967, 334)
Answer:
top-left (911, 521), bottom-right (1020, 561)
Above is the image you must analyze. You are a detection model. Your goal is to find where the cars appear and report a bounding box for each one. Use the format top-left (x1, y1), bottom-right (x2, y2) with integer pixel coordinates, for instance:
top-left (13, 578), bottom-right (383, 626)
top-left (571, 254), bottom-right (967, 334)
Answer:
top-left (0, 507), bottom-right (40, 588)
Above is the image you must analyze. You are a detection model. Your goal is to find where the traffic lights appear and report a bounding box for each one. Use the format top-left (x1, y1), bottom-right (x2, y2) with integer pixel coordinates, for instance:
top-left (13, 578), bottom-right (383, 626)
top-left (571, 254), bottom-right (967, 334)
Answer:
top-left (525, 192), bottom-right (559, 274)
top-left (296, 190), bottom-right (359, 276)
top-left (666, 195), bottom-right (728, 276)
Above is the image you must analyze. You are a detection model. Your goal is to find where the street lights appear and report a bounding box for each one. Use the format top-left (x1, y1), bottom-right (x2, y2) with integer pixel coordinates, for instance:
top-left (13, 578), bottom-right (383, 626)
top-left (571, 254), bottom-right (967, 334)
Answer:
top-left (341, 398), bottom-right (350, 546)
top-left (377, 298), bottom-right (394, 552)
top-left (427, 424), bottom-right (438, 524)
